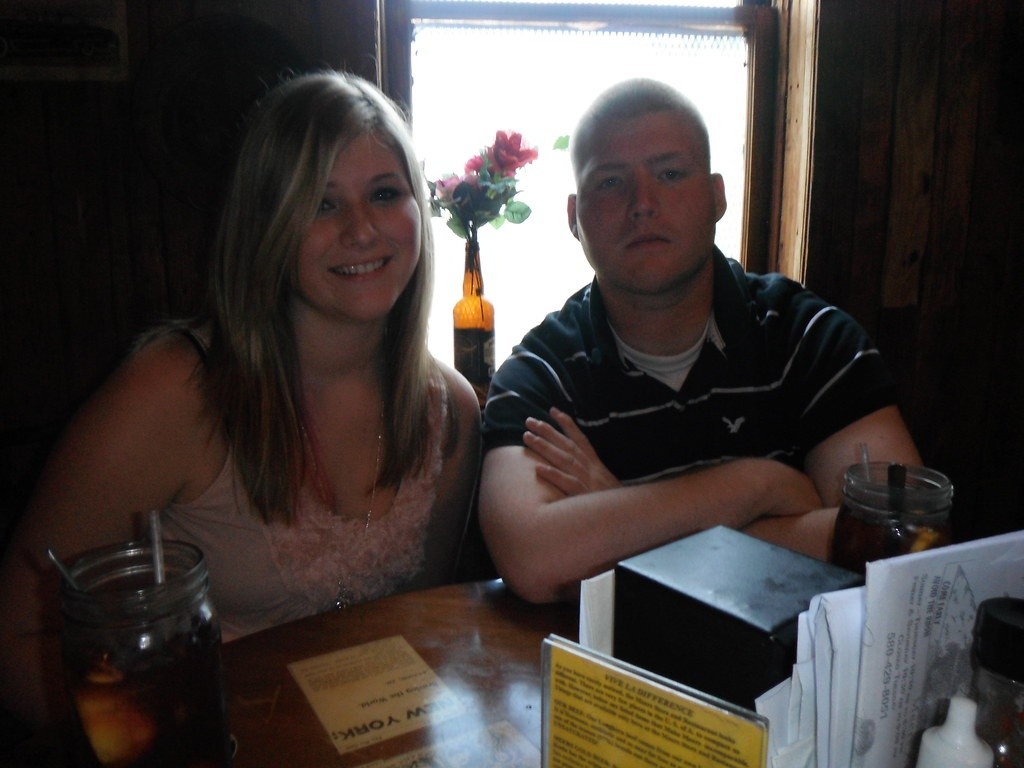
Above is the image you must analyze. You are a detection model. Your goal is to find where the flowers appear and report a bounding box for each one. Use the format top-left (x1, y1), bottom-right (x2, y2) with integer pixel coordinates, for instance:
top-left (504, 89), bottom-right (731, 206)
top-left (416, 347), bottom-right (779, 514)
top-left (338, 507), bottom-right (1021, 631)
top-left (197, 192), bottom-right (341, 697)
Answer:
top-left (421, 129), bottom-right (539, 322)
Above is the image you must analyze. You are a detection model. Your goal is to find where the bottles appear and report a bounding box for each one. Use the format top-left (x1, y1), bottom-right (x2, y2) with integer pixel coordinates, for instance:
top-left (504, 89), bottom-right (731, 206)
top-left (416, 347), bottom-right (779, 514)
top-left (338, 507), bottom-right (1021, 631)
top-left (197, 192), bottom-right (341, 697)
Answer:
top-left (915, 697), bottom-right (993, 768)
top-left (969, 597), bottom-right (1024, 768)
top-left (832, 462), bottom-right (954, 575)
top-left (57, 539), bottom-right (232, 768)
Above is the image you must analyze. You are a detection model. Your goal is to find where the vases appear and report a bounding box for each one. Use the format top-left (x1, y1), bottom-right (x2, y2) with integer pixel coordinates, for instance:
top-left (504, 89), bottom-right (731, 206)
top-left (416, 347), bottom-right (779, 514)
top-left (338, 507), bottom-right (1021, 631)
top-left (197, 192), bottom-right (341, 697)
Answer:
top-left (453, 241), bottom-right (496, 410)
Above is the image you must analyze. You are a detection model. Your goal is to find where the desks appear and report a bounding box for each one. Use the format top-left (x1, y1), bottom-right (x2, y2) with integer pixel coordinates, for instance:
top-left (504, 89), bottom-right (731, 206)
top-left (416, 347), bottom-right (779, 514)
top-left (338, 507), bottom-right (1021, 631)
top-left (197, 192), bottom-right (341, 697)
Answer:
top-left (0, 580), bottom-right (579, 768)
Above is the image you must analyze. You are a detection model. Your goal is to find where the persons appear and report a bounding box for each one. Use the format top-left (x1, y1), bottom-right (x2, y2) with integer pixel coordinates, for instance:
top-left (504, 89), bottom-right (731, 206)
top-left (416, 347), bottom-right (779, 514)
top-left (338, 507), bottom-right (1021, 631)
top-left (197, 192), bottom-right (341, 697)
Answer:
top-left (0, 70), bottom-right (479, 768)
top-left (475, 79), bottom-right (926, 611)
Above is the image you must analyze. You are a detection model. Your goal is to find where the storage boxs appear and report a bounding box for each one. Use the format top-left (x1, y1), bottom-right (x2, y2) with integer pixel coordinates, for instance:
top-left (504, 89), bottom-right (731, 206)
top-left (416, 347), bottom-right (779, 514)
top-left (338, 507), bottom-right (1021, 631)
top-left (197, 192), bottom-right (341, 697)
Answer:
top-left (612, 524), bottom-right (866, 709)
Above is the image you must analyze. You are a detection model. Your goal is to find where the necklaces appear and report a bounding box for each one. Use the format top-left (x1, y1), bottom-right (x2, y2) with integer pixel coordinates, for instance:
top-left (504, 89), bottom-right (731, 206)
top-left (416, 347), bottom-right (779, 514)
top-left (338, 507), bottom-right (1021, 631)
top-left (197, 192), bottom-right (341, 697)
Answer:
top-left (300, 397), bottom-right (384, 609)
